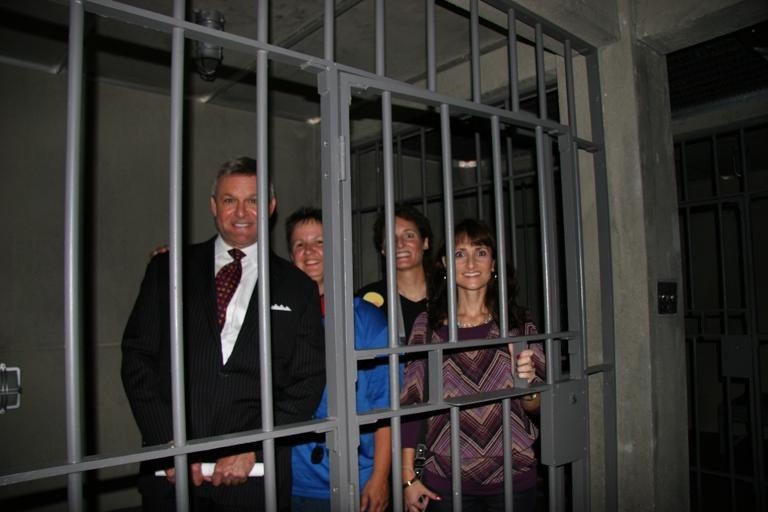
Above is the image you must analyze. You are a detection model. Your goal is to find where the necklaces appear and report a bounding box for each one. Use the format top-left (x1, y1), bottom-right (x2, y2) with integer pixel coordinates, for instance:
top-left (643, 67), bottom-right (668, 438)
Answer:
top-left (457, 314), bottom-right (493, 328)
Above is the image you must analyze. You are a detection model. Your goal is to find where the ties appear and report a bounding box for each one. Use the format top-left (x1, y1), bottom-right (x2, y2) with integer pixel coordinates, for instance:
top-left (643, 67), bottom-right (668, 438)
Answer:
top-left (215, 248), bottom-right (247, 333)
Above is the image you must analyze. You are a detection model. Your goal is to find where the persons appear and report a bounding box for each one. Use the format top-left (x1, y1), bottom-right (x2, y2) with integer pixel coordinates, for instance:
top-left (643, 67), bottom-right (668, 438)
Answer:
top-left (401, 217), bottom-right (550, 512)
top-left (354, 204), bottom-right (435, 342)
top-left (119, 156), bottom-right (326, 511)
top-left (149, 206), bottom-right (405, 512)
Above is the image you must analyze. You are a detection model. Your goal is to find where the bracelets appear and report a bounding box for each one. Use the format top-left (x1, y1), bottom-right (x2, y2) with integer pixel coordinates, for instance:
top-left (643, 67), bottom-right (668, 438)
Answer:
top-left (403, 476), bottom-right (418, 488)
top-left (522, 394), bottom-right (536, 401)
